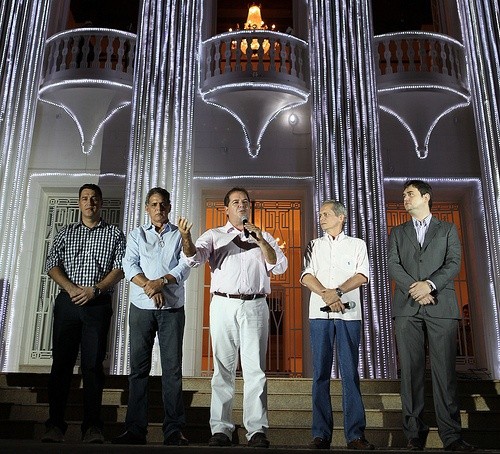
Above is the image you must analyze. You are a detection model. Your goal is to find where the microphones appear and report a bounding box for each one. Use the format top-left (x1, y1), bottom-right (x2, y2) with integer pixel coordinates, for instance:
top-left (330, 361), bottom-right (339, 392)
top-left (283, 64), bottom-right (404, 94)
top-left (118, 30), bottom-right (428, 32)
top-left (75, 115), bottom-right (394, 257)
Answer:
top-left (320, 301), bottom-right (356, 312)
top-left (242, 215), bottom-right (250, 238)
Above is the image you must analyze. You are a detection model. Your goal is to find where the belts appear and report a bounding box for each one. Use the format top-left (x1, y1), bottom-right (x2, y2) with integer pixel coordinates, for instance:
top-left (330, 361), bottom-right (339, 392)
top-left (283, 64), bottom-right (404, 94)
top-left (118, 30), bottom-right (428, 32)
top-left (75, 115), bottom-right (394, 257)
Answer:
top-left (213, 292), bottom-right (267, 301)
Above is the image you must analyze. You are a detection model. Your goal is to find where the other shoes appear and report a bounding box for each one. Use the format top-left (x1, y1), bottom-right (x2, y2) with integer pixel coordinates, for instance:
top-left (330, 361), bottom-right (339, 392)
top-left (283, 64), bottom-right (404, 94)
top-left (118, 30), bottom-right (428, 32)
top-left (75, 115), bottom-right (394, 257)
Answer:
top-left (82, 427), bottom-right (105, 444)
top-left (347, 437), bottom-right (376, 450)
top-left (406, 441), bottom-right (425, 451)
top-left (111, 430), bottom-right (147, 445)
top-left (41, 428), bottom-right (66, 443)
top-left (248, 433), bottom-right (271, 448)
top-left (163, 431), bottom-right (189, 446)
top-left (308, 437), bottom-right (331, 449)
top-left (208, 433), bottom-right (233, 447)
top-left (445, 439), bottom-right (476, 451)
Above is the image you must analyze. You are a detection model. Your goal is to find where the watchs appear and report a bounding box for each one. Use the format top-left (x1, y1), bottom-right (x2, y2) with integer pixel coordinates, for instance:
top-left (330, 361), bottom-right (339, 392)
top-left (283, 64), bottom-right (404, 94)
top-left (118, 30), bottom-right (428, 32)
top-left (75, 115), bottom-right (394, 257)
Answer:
top-left (426, 280), bottom-right (434, 292)
top-left (336, 288), bottom-right (343, 298)
top-left (160, 277), bottom-right (169, 287)
top-left (92, 285), bottom-right (101, 298)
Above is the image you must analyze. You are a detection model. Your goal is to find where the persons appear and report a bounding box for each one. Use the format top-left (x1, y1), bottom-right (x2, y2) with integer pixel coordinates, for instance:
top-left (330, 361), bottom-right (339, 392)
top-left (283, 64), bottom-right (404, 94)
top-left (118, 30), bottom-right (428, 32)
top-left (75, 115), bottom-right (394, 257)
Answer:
top-left (40, 183), bottom-right (125, 444)
top-left (299, 199), bottom-right (376, 450)
top-left (177, 187), bottom-right (288, 448)
top-left (122, 187), bottom-right (191, 447)
top-left (387, 180), bottom-right (479, 451)
